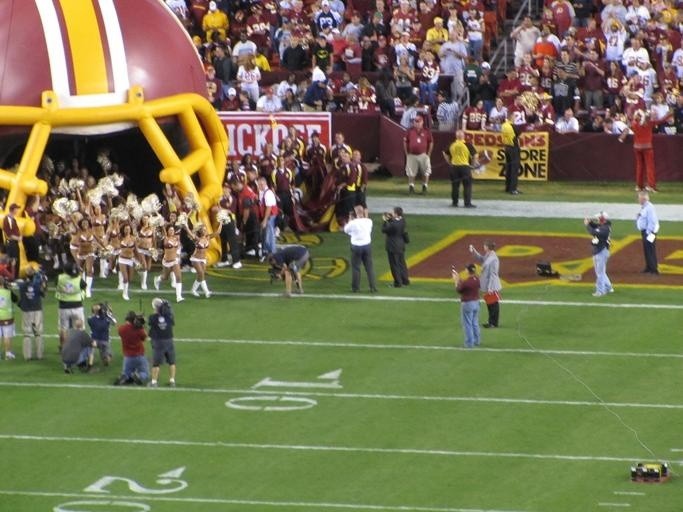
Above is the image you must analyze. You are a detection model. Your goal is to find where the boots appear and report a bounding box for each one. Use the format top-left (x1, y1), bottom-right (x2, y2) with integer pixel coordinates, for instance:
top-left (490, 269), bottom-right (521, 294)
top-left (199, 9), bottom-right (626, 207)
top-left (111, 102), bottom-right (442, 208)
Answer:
top-left (52, 253), bottom-right (147, 301)
top-left (154, 271), bottom-right (213, 303)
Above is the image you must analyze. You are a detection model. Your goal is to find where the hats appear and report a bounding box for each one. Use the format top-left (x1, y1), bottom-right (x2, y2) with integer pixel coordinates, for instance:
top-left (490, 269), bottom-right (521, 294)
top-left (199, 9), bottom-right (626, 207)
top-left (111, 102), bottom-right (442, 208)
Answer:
top-left (464, 263), bottom-right (476, 271)
top-left (228, 88), bottom-right (237, 97)
top-left (10, 204), bottom-right (20, 209)
top-left (321, 0), bottom-right (329, 7)
top-left (595, 211), bottom-right (607, 222)
top-left (434, 17), bottom-right (443, 25)
top-left (209, 1), bottom-right (216, 11)
top-left (374, 12), bottom-right (383, 18)
top-left (266, 87), bottom-right (274, 93)
top-left (415, 116), bottom-right (423, 122)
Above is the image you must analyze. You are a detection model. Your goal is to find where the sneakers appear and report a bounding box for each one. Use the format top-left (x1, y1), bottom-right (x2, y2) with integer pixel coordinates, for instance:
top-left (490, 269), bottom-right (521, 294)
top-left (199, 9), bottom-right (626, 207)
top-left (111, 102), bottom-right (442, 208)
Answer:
top-left (370, 287), bottom-right (378, 293)
top-left (388, 281), bottom-right (409, 288)
top-left (635, 184), bottom-right (657, 193)
top-left (352, 287), bottom-right (357, 293)
top-left (5, 351), bottom-right (16, 360)
top-left (64, 363), bottom-right (96, 374)
top-left (506, 187), bottom-right (522, 194)
top-left (281, 289), bottom-right (303, 298)
top-left (483, 323), bottom-right (496, 328)
top-left (113, 371), bottom-right (175, 387)
top-left (217, 261), bottom-right (242, 269)
top-left (453, 201), bottom-right (475, 208)
top-left (592, 288), bottom-right (614, 296)
top-left (409, 185), bottom-right (427, 197)
top-left (641, 269), bottom-right (658, 274)
top-left (104, 356), bottom-right (111, 365)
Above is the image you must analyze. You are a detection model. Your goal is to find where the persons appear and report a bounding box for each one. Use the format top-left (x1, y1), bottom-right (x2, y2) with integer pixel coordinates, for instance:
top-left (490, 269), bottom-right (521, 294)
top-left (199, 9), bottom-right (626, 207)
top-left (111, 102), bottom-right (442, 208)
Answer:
top-left (1, 124), bottom-right (415, 303)
top-left (441, 129), bottom-right (479, 208)
top-left (0, 262), bottom-right (176, 389)
top-left (469, 237), bottom-right (503, 328)
top-left (164, 0), bottom-right (415, 132)
top-left (402, 114), bottom-right (434, 195)
top-left (416, 1), bottom-right (683, 143)
top-left (583, 211), bottom-right (614, 296)
top-left (449, 262), bottom-right (482, 349)
top-left (500, 112), bottom-right (523, 196)
top-left (634, 191), bottom-right (661, 276)
top-left (631, 106), bottom-right (676, 194)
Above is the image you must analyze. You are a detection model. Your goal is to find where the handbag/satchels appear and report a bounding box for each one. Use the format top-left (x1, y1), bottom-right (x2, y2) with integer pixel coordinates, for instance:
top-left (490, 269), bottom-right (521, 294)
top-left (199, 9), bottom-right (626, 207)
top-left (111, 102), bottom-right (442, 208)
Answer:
top-left (403, 232), bottom-right (410, 243)
